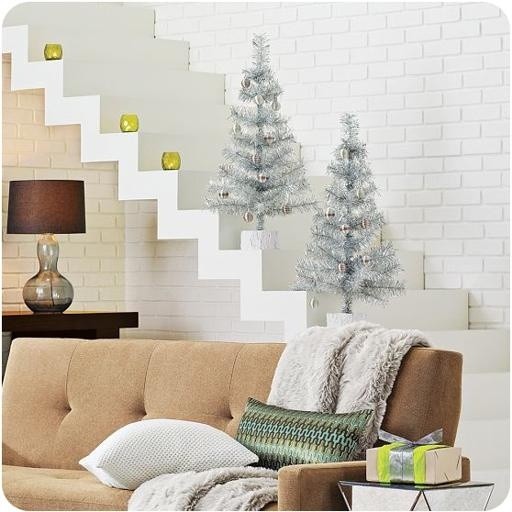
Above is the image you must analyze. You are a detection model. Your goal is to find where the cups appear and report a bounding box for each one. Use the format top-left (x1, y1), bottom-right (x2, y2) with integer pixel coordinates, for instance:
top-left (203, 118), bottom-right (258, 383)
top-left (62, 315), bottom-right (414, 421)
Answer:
top-left (118, 111), bottom-right (140, 132)
top-left (160, 150), bottom-right (182, 170)
top-left (42, 42), bottom-right (65, 61)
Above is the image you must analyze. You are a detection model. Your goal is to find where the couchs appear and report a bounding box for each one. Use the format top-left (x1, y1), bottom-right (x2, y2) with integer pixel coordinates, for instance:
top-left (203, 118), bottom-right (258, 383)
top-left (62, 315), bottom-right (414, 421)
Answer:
top-left (0, 324), bottom-right (461, 511)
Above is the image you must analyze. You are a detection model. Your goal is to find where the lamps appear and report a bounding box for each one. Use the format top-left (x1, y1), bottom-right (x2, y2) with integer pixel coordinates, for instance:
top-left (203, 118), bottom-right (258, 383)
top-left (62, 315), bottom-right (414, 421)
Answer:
top-left (6, 179), bottom-right (86, 314)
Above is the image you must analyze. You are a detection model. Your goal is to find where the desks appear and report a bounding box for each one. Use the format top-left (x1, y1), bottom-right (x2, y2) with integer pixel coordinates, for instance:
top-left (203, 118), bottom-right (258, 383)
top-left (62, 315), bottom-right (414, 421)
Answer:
top-left (1, 311), bottom-right (137, 339)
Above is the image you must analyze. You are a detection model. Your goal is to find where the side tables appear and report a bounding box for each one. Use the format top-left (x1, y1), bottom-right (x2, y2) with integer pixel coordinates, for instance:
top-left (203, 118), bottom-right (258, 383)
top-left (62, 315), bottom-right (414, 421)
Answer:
top-left (334, 479), bottom-right (497, 509)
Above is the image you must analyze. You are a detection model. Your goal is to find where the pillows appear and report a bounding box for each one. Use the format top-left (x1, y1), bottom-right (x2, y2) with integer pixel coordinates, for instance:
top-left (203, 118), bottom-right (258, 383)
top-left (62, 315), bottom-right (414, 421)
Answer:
top-left (78, 398), bottom-right (374, 502)
